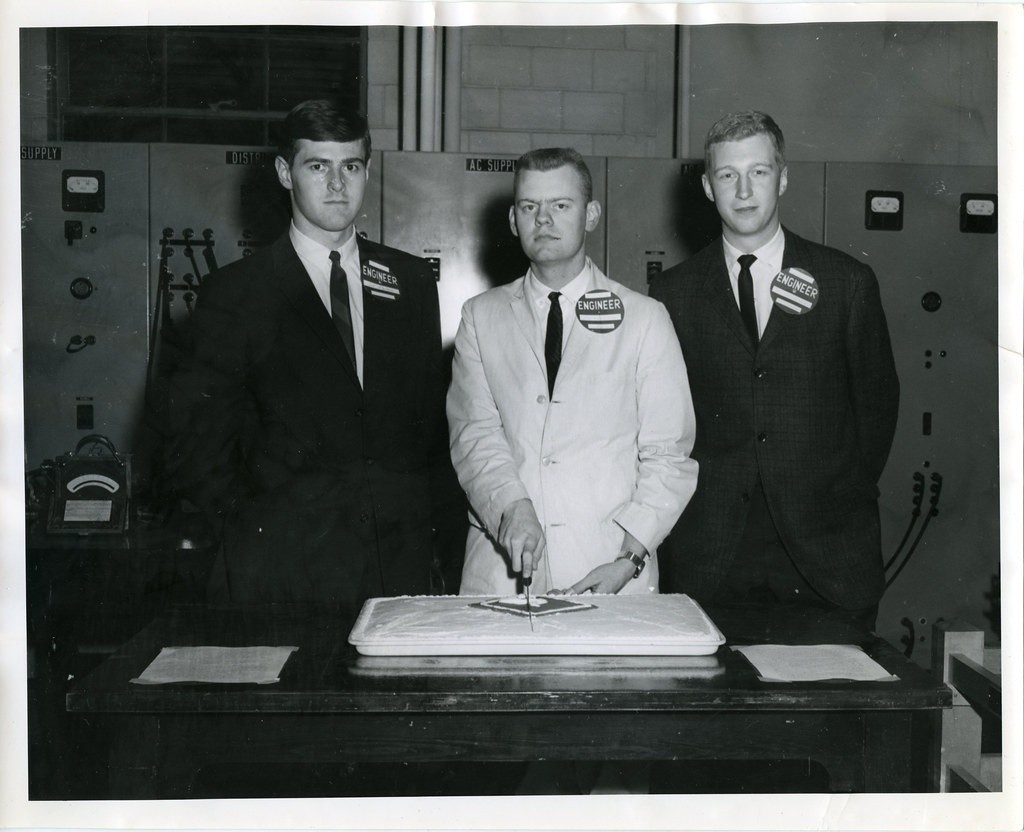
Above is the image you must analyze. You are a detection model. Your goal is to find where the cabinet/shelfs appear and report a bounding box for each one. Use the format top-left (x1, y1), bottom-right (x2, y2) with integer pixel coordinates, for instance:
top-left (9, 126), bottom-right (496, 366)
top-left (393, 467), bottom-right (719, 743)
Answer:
top-left (932, 617), bottom-right (1002, 793)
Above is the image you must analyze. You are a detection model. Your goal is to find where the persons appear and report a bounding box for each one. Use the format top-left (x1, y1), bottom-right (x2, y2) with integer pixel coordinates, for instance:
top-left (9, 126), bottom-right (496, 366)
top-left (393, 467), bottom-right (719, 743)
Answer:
top-left (446, 147), bottom-right (700, 595)
top-left (143, 101), bottom-right (451, 641)
top-left (645, 112), bottom-right (900, 643)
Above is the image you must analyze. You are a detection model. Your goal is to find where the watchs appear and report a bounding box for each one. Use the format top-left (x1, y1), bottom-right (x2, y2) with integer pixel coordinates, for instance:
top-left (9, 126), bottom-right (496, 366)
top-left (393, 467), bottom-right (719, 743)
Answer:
top-left (615, 550), bottom-right (645, 580)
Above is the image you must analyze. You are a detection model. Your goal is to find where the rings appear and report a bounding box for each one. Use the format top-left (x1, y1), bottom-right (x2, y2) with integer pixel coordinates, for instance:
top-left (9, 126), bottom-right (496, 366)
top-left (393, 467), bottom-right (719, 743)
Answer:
top-left (523, 545), bottom-right (534, 553)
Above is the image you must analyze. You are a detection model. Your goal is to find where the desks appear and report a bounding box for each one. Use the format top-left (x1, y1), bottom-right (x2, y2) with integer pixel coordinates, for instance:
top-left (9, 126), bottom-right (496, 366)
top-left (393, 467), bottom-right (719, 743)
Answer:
top-left (64, 604), bottom-right (953, 800)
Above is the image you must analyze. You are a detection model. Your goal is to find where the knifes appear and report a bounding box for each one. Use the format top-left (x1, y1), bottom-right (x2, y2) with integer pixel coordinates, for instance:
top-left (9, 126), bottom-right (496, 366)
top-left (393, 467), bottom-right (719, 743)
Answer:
top-left (522, 576), bottom-right (534, 632)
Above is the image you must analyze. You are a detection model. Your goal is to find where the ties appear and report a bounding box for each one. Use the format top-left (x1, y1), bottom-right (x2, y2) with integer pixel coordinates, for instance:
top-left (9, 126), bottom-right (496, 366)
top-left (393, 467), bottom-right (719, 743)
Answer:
top-left (542, 291), bottom-right (563, 402)
top-left (735, 254), bottom-right (760, 351)
top-left (326, 249), bottom-right (358, 375)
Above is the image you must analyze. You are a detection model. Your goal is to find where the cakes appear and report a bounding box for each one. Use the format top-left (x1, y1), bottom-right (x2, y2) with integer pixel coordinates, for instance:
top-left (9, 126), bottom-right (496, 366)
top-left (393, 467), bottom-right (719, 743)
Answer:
top-left (350, 592), bottom-right (721, 643)
